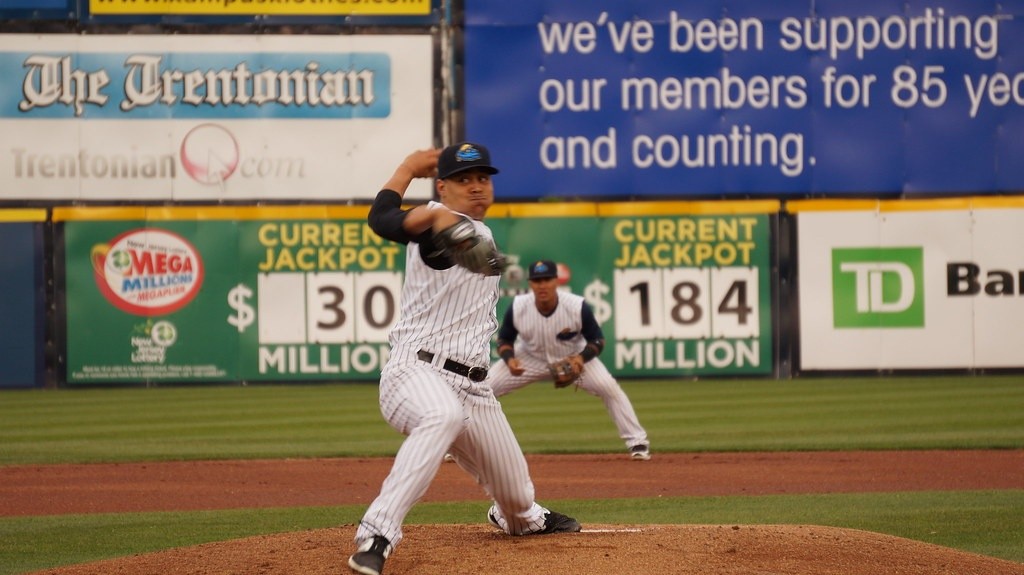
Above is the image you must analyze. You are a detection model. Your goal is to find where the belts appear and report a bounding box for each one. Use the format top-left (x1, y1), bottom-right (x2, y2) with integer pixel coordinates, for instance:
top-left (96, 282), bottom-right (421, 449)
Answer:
top-left (417, 350), bottom-right (487, 383)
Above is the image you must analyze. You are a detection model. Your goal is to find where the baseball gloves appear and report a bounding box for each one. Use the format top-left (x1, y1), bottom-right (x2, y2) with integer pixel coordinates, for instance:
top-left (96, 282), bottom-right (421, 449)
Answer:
top-left (431, 212), bottom-right (517, 281)
top-left (547, 355), bottom-right (585, 393)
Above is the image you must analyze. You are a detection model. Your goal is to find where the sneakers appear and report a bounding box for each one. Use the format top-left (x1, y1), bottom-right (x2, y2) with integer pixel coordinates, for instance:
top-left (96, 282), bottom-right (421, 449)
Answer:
top-left (488, 501), bottom-right (582, 534)
top-left (445, 453), bottom-right (455, 462)
top-left (348, 519), bottom-right (393, 575)
top-left (631, 440), bottom-right (651, 460)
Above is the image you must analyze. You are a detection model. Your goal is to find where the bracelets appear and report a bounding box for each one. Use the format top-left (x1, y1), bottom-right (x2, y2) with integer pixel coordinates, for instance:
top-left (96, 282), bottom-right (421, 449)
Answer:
top-left (579, 345), bottom-right (598, 363)
top-left (500, 349), bottom-right (515, 366)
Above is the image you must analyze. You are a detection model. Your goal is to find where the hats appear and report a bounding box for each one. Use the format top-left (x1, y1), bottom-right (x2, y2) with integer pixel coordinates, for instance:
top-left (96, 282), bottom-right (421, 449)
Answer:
top-left (439, 142), bottom-right (499, 180)
top-left (529, 261), bottom-right (557, 280)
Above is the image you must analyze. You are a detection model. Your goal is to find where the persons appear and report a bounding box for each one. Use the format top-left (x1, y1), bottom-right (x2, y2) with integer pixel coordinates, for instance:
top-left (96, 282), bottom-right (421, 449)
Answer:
top-left (347, 144), bottom-right (583, 575)
top-left (443, 260), bottom-right (651, 463)
